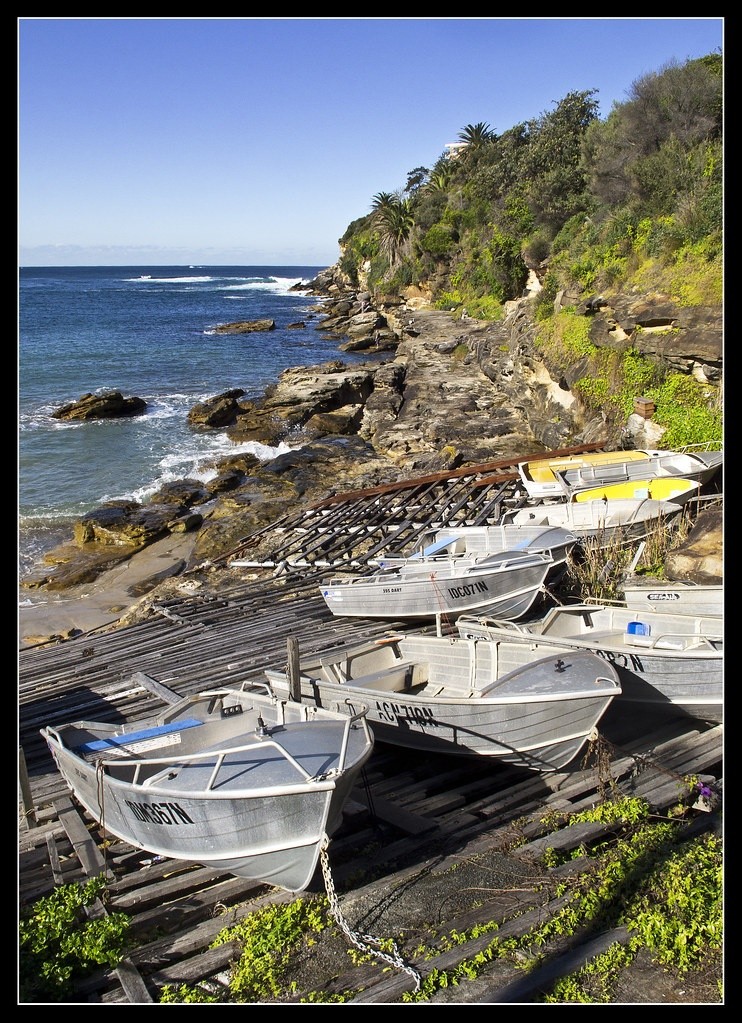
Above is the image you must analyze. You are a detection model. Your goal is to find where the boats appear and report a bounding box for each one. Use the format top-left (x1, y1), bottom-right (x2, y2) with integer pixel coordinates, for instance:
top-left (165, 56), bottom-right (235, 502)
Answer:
top-left (500, 497), bottom-right (686, 552)
top-left (369, 527), bottom-right (579, 575)
top-left (319, 543), bottom-right (555, 622)
top-left (555, 441), bottom-right (723, 500)
top-left (262, 630), bottom-right (624, 771)
top-left (568, 477), bottom-right (704, 509)
top-left (622, 579), bottom-right (724, 618)
top-left (518, 450), bottom-right (682, 499)
top-left (455, 597), bottom-right (724, 727)
top-left (39, 679), bottom-right (376, 893)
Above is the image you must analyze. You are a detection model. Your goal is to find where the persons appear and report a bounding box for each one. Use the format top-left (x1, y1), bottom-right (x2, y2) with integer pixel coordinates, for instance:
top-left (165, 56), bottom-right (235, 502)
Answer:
top-left (375, 330), bottom-right (380, 349)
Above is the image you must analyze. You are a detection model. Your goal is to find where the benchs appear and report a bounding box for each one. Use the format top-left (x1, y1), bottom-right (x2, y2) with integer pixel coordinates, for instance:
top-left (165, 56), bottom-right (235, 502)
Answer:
top-left (80, 718), bottom-right (203, 756)
top-left (608, 511), bottom-right (637, 524)
top-left (412, 535), bottom-right (463, 561)
top-left (343, 661), bottom-right (422, 687)
top-left (509, 536), bottom-right (532, 552)
top-left (563, 629), bottom-right (627, 640)
top-left (662, 466), bottom-right (683, 475)
